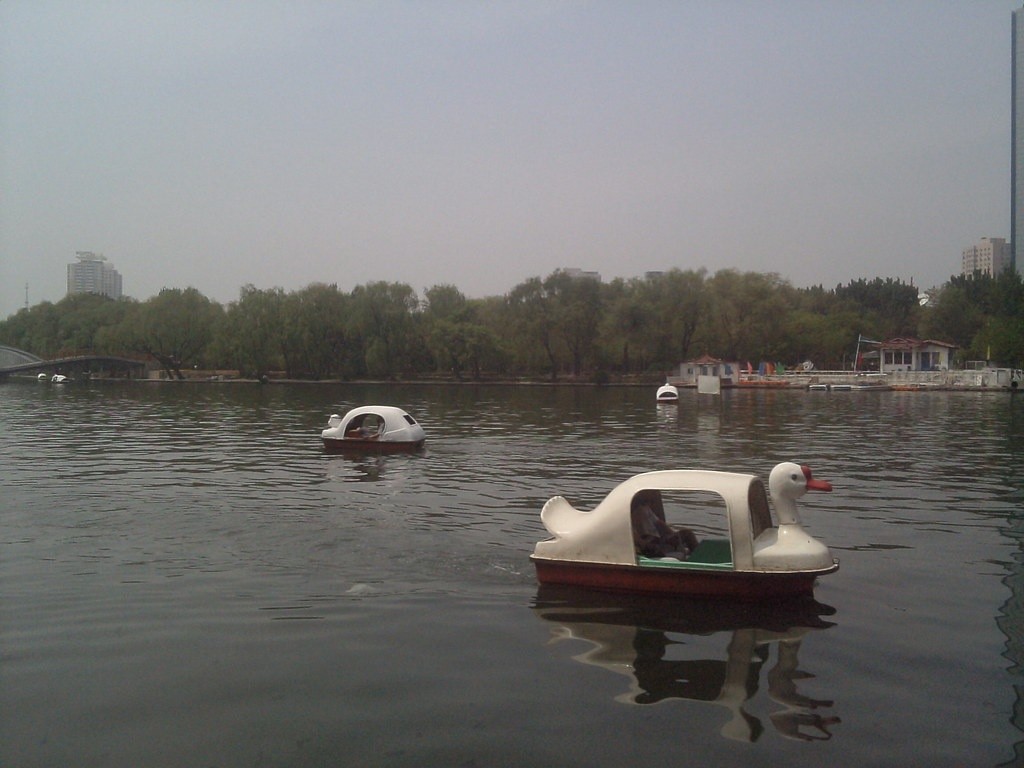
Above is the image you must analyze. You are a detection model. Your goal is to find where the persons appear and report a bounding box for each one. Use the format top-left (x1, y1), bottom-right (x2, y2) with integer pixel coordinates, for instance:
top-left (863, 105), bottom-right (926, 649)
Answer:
top-left (631, 491), bottom-right (699, 557)
top-left (362, 416), bottom-right (385, 438)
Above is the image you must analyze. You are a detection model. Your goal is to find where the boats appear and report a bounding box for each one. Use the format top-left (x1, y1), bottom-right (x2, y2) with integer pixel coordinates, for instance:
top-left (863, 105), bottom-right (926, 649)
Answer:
top-left (656, 382), bottom-right (679, 404)
top-left (320, 405), bottom-right (426, 453)
top-left (810, 372), bottom-right (920, 393)
top-left (37, 373), bottom-right (47, 380)
top-left (51, 374), bottom-right (70, 383)
top-left (527, 461), bottom-right (842, 607)
